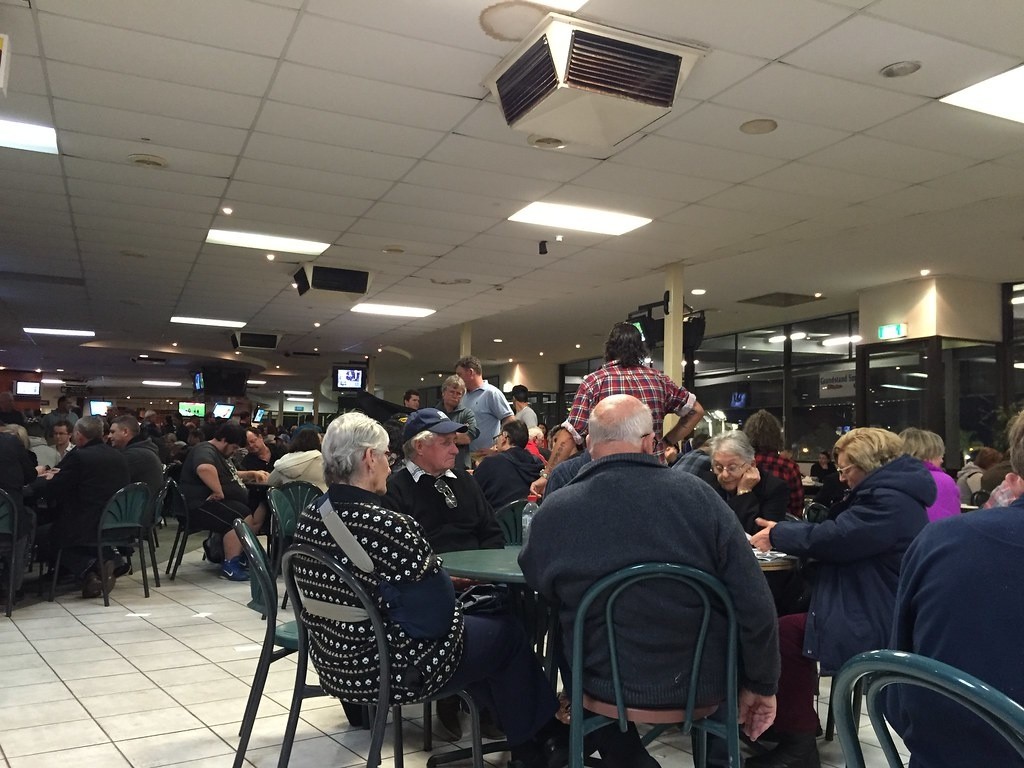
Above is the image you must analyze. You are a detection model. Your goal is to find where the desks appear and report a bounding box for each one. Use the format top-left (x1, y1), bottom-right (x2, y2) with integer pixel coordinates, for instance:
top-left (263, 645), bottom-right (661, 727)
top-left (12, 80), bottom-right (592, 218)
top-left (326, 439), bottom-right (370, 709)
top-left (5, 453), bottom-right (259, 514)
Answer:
top-left (427, 545), bottom-right (803, 768)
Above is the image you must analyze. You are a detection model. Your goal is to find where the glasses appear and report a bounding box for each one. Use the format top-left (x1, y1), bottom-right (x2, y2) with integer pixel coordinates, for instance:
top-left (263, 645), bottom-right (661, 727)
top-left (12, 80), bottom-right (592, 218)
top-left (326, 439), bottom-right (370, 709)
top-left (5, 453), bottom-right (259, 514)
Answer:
top-left (492, 433), bottom-right (510, 442)
top-left (362, 448), bottom-right (396, 466)
top-left (836, 463), bottom-right (857, 476)
top-left (641, 434), bottom-right (658, 453)
top-left (709, 460), bottom-right (747, 474)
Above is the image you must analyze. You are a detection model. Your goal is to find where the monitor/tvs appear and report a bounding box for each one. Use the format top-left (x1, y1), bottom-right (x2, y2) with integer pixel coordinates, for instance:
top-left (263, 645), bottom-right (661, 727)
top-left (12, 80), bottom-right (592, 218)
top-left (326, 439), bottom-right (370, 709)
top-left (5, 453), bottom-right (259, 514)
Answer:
top-left (192, 373), bottom-right (204, 390)
top-left (626, 315), bottom-right (652, 347)
top-left (90, 400), bottom-right (113, 417)
top-left (332, 365), bottom-right (366, 391)
top-left (212, 402), bottom-right (235, 419)
top-left (178, 402), bottom-right (206, 418)
top-left (252, 408), bottom-right (265, 423)
top-left (16, 380), bottom-right (41, 396)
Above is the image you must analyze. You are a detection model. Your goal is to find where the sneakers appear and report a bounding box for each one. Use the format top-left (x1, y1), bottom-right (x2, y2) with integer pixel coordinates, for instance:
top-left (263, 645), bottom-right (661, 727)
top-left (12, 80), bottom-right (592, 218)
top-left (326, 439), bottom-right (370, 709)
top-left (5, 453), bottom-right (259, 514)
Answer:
top-left (236, 555), bottom-right (248, 570)
top-left (219, 557), bottom-right (250, 581)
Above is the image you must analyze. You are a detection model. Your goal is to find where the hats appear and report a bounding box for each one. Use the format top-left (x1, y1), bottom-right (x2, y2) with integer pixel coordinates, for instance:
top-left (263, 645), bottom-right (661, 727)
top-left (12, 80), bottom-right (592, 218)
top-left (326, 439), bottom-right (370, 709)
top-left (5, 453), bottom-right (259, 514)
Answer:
top-left (401, 407), bottom-right (468, 445)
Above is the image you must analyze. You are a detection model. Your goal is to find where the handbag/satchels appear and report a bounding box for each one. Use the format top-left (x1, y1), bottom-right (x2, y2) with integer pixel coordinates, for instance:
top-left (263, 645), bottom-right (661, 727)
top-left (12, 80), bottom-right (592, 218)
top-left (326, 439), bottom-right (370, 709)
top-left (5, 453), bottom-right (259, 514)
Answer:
top-left (784, 578), bottom-right (814, 615)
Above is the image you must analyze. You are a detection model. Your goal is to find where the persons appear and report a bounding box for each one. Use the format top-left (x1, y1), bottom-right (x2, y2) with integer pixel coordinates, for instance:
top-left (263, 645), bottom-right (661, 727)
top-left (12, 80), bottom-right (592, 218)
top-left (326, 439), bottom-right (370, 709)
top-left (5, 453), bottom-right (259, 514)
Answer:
top-left (382, 355), bottom-right (592, 739)
top-left (663, 406), bottom-right (1024, 768)
top-left (529, 322), bottom-right (704, 499)
top-left (518, 395), bottom-right (783, 768)
top-left (289, 411), bottom-right (571, 768)
top-left (0, 392), bottom-right (371, 598)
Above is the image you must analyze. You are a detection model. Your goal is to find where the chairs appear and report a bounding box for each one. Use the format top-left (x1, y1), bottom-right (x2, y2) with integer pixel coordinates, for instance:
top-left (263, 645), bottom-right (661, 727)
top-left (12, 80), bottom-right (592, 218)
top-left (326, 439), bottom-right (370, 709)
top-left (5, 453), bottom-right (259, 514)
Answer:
top-left (0, 459), bottom-right (1024, 768)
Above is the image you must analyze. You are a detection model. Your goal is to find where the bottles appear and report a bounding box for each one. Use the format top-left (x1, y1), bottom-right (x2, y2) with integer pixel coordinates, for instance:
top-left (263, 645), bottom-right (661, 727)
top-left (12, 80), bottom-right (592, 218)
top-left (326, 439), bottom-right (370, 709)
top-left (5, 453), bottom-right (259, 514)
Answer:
top-left (521, 495), bottom-right (540, 552)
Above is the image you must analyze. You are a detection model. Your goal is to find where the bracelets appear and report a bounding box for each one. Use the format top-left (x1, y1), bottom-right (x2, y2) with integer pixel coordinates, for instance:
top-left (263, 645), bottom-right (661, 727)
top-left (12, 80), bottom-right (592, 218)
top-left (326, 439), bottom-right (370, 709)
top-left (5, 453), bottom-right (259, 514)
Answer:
top-left (736, 489), bottom-right (751, 496)
top-left (663, 436), bottom-right (672, 447)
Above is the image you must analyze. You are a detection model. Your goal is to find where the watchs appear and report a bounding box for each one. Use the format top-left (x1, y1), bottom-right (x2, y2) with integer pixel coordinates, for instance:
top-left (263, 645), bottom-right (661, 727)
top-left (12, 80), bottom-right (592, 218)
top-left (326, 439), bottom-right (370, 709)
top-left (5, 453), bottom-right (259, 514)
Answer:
top-left (540, 470), bottom-right (550, 479)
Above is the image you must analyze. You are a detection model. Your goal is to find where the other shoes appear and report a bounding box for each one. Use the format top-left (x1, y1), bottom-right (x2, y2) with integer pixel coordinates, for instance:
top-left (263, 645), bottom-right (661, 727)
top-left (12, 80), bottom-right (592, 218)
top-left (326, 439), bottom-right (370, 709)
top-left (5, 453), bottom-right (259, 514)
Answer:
top-left (82, 571), bottom-right (102, 598)
top-left (758, 724), bottom-right (822, 742)
top-left (479, 714), bottom-right (506, 740)
top-left (96, 558), bottom-right (116, 595)
top-left (0, 586), bottom-right (25, 605)
top-left (436, 701), bottom-right (462, 740)
top-left (202, 538), bottom-right (221, 563)
top-left (113, 561), bottom-right (130, 578)
top-left (744, 741), bottom-right (821, 768)
